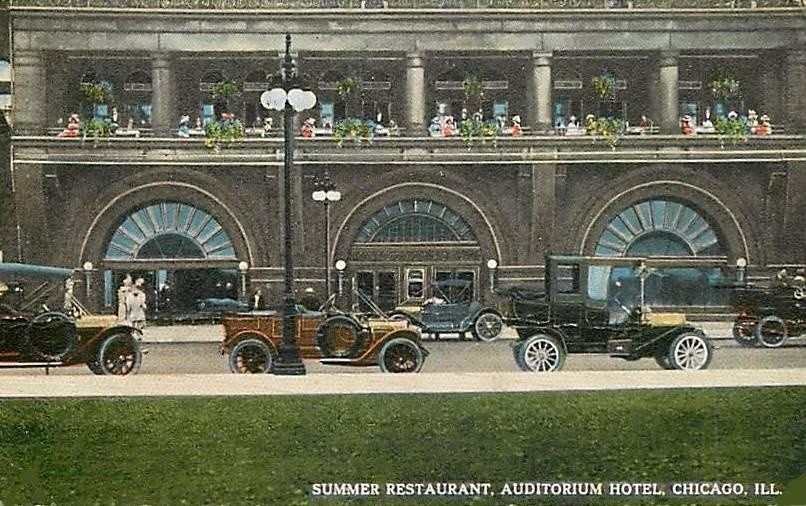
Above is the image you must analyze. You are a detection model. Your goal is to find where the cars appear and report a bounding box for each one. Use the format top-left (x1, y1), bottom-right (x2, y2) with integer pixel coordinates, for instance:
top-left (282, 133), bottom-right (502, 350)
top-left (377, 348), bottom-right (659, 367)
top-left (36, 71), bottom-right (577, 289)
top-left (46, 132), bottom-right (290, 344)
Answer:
top-left (1, 262), bottom-right (142, 376)
top-left (508, 255), bottom-right (714, 372)
top-left (727, 283), bottom-right (801, 349)
top-left (218, 279), bottom-right (505, 372)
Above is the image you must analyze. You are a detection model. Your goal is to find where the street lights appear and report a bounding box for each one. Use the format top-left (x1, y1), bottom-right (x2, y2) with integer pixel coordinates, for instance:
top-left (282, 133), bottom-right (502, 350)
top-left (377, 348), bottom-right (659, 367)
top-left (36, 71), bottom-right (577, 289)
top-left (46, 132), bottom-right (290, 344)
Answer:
top-left (737, 258), bottom-right (747, 283)
top-left (261, 29), bottom-right (317, 375)
top-left (334, 260), bottom-right (349, 297)
top-left (488, 259), bottom-right (499, 293)
top-left (312, 172), bottom-right (342, 307)
top-left (238, 261), bottom-right (252, 297)
